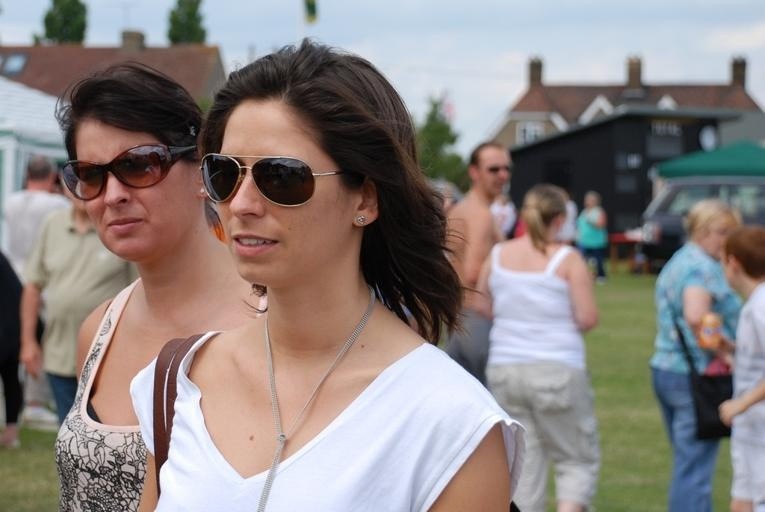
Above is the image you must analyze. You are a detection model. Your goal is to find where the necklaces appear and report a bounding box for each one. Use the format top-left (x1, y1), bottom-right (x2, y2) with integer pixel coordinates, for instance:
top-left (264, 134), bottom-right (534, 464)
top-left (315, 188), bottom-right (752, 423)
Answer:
top-left (259, 282), bottom-right (379, 512)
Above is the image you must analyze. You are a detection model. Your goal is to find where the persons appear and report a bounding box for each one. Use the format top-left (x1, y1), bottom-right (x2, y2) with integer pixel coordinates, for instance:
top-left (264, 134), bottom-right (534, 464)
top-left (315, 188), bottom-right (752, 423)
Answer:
top-left (52, 60), bottom-right (268, 512)
top-left (20, 161), bottom-right (141, 430)
top-left (649, 197), bottom-right (747, 512)
top-left (2, 153), bottom-right (73, 426)
top-left (577, 185), bottom-right (609, 281)
top-left (129, 34), bottom-right (528, 512)
top-left (697, 224), bottom-right (764, 512)
top-left (553, 187), bottom-right (580, 244)
top-left (486, 188), bottom-right (519, 240)
top-left (474, 183), bottom-right (602, 512)
top-left (0, 250), bottom-right (43, 448)
top-left (440, 140), bottom-right (512, 392)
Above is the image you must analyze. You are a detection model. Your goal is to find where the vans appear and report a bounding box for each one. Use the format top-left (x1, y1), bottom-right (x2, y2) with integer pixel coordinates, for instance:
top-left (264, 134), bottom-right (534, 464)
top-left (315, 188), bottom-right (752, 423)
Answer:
top-left (641, 175), bottom-right (765, 261)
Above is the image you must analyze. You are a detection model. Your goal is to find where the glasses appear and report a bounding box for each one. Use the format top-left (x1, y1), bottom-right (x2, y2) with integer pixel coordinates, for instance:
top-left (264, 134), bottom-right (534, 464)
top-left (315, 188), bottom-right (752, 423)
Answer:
top-left (200, 156), bottom-right (340, 208)
top-left (480, 163), bottom-right (511, 177)
top-left (61, 146), bottom-right (200, 202)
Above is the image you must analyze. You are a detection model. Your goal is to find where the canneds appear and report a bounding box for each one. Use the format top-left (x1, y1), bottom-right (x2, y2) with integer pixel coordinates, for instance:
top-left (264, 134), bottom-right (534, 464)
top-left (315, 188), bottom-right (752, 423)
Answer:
top-left (698, 312), bottom-right (723, 350)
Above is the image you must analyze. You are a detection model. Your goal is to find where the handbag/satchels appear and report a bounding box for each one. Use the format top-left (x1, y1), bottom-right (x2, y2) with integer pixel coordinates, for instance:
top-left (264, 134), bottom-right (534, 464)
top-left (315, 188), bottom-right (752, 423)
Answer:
top-left (690, 373), bottom-right (734, 439)
